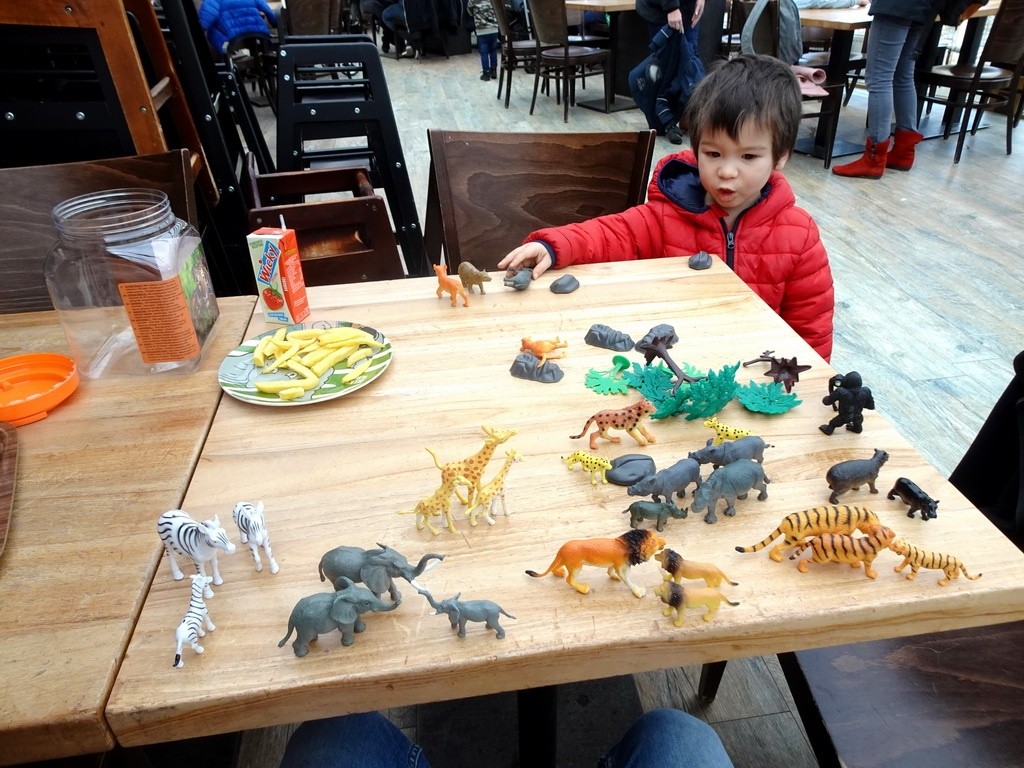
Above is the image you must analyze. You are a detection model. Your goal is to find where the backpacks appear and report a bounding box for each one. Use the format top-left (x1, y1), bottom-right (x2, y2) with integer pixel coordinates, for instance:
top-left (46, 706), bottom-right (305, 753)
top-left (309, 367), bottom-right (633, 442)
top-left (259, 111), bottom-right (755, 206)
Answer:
top-left (741, 1), bottom-right (803, 68)
top-left (938, 0), bottom-right (990, 29)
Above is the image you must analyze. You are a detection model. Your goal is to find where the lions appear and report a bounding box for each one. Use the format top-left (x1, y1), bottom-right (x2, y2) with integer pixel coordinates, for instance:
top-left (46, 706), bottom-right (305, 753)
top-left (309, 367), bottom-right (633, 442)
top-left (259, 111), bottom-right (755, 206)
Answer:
top-left (654, 549), bottom-right (738, 590)
top-left (654, 579), bottom-right (740, 627)
top-left (525, 529), bottom-right (666, 599)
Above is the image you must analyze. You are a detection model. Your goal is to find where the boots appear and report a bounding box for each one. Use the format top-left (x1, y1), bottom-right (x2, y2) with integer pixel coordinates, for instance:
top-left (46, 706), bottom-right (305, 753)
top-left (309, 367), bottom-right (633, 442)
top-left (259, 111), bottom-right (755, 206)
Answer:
top-left (885, 126), bottom-right (923, 172)
top-left (490, 67), bottom-right (498, 79)
top-left (832, 136), bottom-right (889, 178)
top-left (479, 69), bottom-right (490, 81)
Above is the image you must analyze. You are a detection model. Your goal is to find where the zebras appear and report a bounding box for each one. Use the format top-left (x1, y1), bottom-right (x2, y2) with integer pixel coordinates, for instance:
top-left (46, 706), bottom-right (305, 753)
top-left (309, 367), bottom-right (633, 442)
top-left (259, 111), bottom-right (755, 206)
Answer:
top-left (171, 574), bottom-right (213, 669)
top-left (232, 501), bottom-right (280, 575)
top-left (157, 509), bottom-right (236, 599)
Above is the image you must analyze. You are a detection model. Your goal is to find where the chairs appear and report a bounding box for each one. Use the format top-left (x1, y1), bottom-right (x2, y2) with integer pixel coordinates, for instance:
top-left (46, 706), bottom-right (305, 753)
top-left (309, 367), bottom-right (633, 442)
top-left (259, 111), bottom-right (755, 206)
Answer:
top-left (1, 147), bottom-right (201, 314)
top-left (797, 20), bottom-right (869, 129)
top-left (734, 0), bottom-right (846, 169)
top-left (540, 4), bottom-right (612, 97)
top-left (284, 0), bottom-right (341, 82)
top-left (225, 29), bottom-right (281, 106)
top-left (391, 0), bottom-right (449, 64)
top-left (841, 25), bottom-right (952, 116)
top-left (242, 151), bottom-right (405, 288)
top-left (268, 33), bottom-right (424, 278)
top-left (489, 0), bottom-right (563, 108)
top-left (528, 0), bottom-right (615, 124)
top-left (419, 129), bottom-right (658, 276)
top-left (719, 0), bottom-right (758, 62)
top-left (330, 0), bottom-right (356, 81)
top-left (914, 0), bottom-right (1024, 164)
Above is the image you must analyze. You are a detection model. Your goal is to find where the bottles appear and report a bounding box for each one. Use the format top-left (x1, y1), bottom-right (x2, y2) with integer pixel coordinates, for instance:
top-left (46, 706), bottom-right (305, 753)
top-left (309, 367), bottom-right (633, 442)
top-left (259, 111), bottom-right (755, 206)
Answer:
top-left (42, 187), bottom-right (219, 380)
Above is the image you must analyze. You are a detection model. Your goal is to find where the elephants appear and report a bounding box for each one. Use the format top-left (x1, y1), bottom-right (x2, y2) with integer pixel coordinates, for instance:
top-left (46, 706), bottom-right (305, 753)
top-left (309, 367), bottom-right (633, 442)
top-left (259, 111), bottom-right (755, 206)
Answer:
top-left (418, 589), bottom-right (516, 641)
top-left (277, 542), bottom-right (445, 658)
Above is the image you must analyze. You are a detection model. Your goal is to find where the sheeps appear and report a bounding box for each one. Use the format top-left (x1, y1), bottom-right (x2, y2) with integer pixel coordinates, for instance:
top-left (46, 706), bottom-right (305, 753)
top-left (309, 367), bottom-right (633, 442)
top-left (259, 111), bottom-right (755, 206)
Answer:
top-left (826, 449), bottom-right (888, 505)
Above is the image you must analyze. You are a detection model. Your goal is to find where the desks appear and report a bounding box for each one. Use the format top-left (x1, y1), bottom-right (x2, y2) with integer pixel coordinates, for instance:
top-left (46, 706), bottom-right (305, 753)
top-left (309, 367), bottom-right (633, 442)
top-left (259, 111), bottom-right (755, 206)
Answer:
top-left (1, 293), bottom-right (261, 768)
top-left (566, 0), bottom-right (639, 114)
top-left (103, 253), bottom-right (1024, 767)
top-left (792, 0), bottom-right (1004, 161)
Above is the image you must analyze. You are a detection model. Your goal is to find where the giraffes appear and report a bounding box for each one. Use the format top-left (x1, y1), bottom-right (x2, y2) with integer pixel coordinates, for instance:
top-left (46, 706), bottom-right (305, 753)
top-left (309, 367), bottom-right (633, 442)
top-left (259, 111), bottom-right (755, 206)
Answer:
top-left (399, 425), bottom-right (526, 536)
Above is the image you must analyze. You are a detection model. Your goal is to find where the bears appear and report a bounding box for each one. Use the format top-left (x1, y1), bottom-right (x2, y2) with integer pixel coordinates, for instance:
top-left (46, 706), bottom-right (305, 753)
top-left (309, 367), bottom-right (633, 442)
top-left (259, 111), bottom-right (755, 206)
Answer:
top-left (458, 261), bottom-right (492, 295)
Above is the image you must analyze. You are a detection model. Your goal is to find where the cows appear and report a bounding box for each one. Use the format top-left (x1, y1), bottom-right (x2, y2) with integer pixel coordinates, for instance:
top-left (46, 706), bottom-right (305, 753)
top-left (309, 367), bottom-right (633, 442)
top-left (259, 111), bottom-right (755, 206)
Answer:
top-left (888, 477), bottom-right (940, 521)
top-left (503, 266), bottom-right (534, 290)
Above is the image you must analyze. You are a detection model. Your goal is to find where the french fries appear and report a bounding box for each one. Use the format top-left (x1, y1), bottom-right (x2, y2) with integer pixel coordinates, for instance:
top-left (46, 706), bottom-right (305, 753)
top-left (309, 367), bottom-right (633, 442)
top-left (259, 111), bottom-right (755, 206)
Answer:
top-left (253, 328), bottom-right (386, 399)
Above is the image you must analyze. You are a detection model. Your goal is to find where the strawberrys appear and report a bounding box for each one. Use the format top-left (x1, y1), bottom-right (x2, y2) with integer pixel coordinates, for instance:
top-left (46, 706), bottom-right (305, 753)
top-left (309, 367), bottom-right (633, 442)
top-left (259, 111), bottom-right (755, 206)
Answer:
top-left (263, 282), bottom-right (284, 310)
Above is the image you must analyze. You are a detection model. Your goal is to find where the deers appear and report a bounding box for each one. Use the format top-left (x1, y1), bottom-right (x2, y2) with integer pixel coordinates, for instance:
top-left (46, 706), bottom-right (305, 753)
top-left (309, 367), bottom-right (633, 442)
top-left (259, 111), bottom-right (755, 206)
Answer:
top-left (433, 264), bottom-right (469, 308)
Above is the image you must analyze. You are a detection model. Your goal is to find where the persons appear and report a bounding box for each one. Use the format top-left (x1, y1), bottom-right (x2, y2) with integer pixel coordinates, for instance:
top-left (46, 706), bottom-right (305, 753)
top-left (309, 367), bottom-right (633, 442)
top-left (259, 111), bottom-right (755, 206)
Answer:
top-left (467, 0), bottom-right (499, 81)
top-left (199, 0), bottom-right (427, 92)
top-left (276, 707), bottom-right (734, 767)
top-left (509, 0), bottom-right (871, 72)
top-left (496, 53), bottom-right (836, 367)
top-left (635, 0), bottom-right (708, 135)
top-left (832, 0), bottom-right (942, 179)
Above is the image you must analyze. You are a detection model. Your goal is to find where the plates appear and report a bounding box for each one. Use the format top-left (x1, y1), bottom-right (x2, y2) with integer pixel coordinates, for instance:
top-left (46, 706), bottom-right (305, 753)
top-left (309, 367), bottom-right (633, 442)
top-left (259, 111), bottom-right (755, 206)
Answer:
top-left (216, 320), bottom-right (393, 406)
